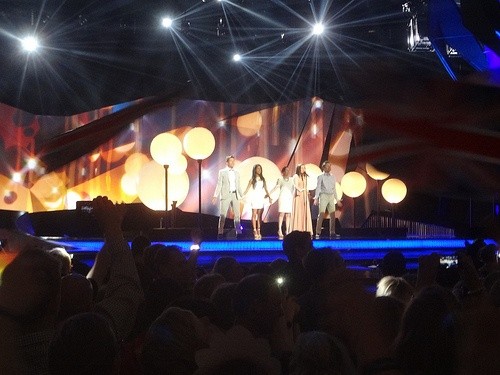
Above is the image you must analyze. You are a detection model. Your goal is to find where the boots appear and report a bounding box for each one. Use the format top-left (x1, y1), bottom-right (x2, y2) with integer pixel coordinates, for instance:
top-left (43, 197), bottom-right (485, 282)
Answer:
top-left (252, 229), bottom-right (261, 240)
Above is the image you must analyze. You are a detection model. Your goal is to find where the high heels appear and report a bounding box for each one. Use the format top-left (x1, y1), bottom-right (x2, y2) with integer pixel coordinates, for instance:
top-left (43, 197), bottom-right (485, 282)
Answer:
top-left (277, 230), bottom-right (284, 240)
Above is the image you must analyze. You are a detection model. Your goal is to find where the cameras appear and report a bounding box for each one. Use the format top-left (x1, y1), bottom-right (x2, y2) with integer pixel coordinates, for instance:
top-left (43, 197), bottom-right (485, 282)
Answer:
top-left (76, 201), bottom-right (94, 213)
top-left (440, 255), bottom-right (459, 269)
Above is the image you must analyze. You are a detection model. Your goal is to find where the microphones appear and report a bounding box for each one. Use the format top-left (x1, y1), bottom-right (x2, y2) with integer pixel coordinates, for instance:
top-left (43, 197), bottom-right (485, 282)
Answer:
top-left (304, 172), bottom-right (308, 176)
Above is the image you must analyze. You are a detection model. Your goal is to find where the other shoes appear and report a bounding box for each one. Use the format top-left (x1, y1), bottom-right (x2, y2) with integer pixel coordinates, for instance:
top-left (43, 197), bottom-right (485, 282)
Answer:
top-left (217, 234), bottom-right (223, 240)
top-left (315, 235), bottom-right (321, 240)
top-left (236, 234), bottom-right (244, 239)
top-left (328, 234), bottom-right (341, 240)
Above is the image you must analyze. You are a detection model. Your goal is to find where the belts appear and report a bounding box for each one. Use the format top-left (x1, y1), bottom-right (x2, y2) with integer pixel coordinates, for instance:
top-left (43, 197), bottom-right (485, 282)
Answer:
top-left (228, 191), bottom-right (236, 194)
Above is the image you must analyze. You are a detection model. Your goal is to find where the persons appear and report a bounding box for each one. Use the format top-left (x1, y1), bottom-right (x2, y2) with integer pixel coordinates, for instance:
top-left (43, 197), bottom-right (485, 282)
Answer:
top-left (289, 164), bottom-right (314, 239)
top-left (240, 164), bottom-right (273, 241)
top-left (312, 161), bottom-right (341, 240)
top-left (0, 195), bottom-right (499, 374)
top-left (211, 155), bottom-right (243, 237)
top-left (264, 166), bottom-right (307, 240)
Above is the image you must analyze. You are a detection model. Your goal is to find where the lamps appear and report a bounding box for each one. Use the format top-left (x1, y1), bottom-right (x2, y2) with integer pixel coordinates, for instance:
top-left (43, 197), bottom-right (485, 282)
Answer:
top-left (182, 127), bottom-right (216, 214)
top-left (150, 132), bottom-right (182, 230)
top-left (381, 178), bottom-right (408, 229)
top-left (366, 163), bottom-right (390, 229)
top-left (340, 172), bottom-right (367, 227)
